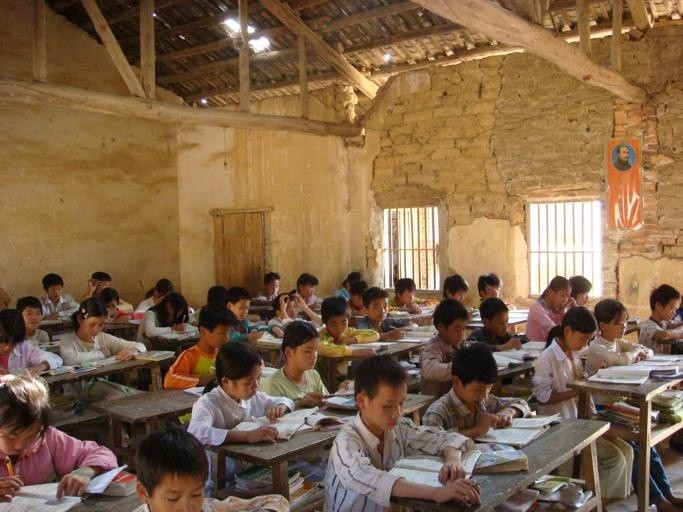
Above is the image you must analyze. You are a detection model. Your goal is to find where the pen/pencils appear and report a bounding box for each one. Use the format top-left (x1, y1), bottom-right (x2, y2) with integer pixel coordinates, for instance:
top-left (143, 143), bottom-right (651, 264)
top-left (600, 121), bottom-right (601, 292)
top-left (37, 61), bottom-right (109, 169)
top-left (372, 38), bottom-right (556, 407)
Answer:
top-left (389, 325), bottom-right (397, 330)
top-left (251, 416), bottom-right (263, 427)
top-left (479, 401), bottom-right (486, 412)
top-left (182, 315), bottom-right (186, 322)
top-left (92, 336), bottom-right (101, 350)
top-left (450, 482), bottom-right (480, 507)
top-left (3, 456), bottom-right (14, 477)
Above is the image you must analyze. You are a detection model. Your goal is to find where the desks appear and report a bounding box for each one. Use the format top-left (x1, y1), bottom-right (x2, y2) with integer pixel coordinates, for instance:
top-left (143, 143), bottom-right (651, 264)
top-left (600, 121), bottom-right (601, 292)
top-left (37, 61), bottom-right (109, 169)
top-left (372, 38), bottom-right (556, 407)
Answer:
top-left (565, 354), bottom-right (683, 512)
top-left (620, 317), bottom-right (640, 342)
top-left (88, 401), bottom-right (200, 466)
top-left (655, 338), bottom-right (683, 354)
top-left (64, 471), bottom-right (143, 512)
top-left (208, 393), bottom-right (435, 511)
top-left (388, 418), bottom-right (611, 511)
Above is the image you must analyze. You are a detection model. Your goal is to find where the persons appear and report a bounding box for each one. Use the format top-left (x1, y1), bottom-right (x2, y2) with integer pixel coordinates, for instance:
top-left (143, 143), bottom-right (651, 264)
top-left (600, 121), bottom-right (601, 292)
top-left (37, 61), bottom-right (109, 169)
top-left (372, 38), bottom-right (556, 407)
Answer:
top-left (1, 270), bottom-right (682, 512)
top-left (615, 146), bottom-right (631, 171)
